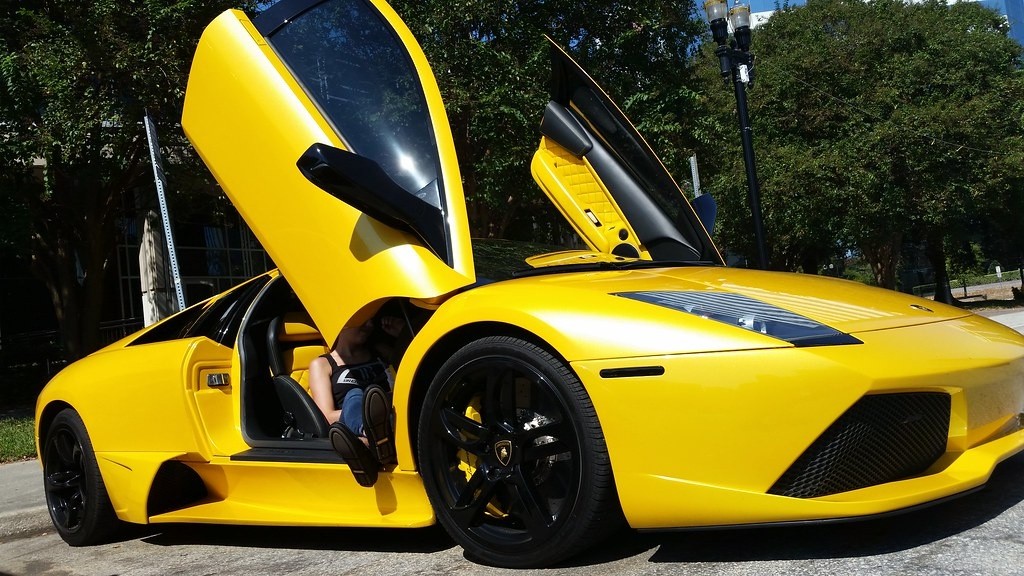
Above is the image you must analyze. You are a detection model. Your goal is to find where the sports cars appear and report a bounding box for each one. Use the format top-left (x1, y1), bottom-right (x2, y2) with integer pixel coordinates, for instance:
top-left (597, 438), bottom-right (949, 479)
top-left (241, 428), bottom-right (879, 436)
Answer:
top-left (34, 0), bottom-right (1024, 569)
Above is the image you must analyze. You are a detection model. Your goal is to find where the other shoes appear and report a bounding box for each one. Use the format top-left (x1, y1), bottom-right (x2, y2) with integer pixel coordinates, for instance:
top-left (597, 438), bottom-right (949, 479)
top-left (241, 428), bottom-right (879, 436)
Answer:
top-left (362, 384), bottom-right (398, 472)
top-left (329, 421), bottom-right (378, 488)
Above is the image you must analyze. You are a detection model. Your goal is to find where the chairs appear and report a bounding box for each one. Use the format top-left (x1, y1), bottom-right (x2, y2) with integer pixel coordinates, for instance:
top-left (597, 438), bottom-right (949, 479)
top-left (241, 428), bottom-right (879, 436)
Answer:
top-left (267, 306), bottom-right (331, 440)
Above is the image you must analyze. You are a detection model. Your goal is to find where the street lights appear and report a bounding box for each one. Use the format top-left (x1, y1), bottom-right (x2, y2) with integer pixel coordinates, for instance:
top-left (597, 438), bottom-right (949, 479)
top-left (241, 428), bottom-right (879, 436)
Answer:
top-left (703, 1), bottom-right (770, 269)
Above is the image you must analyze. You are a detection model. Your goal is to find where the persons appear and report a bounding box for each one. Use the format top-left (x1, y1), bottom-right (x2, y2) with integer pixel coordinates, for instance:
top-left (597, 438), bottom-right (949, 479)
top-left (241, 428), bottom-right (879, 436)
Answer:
top-left (308, 316), bottom-right (396, 487)
